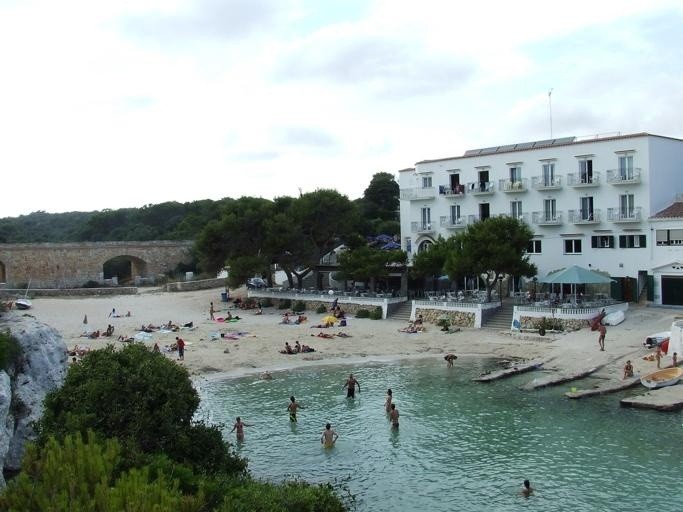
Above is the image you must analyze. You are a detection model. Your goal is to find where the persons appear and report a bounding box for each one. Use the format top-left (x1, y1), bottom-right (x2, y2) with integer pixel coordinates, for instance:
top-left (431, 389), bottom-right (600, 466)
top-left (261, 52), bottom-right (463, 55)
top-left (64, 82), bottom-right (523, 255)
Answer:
top-left (388, 403), bottom-right (400, 428)
top-left (442, 353), bottom-right (458, 369)
top-left (309, 331), bottom-right (332, 339)
top-left (285, 395), bottom-right (308, 423)
top-left (653, 346), bottom-right (663, 369)
top-left (275, 296), bottom-right (347, 329)
top-left (225, 294), bottom-right (264, 316)
top-left (334, 331), bottom-right (353, 338)
top-left (340, 373), bottom-right (361, 399)
top-left (621, 359), bottom-right (633, 380)
top-left (519, 479), bottom-right (537, 495)
top-left (553, 293), bottom-right (560, 305)
top-left (66, 308), bottom-right (197, 366)
top-left (381, 387), bottom-right (394, 412)
top-left (641, 354), bottom-right (654, 361)
top-left (396, 314), bottom-right (425, 334)
top-left (669, 352), bottom-right (677, 367)
top-left (278, 339), bottom-right (313, 355)
top-left (228, 415), bottom-right (256, 441)
top-left (208, 301), bottom-right (216, 321)
top-left (319, 423), bottom-right (339, 450)
top-left (596, 322), bottom-right (606, 351)
top-left (223, 310), bottom-right (231, 321)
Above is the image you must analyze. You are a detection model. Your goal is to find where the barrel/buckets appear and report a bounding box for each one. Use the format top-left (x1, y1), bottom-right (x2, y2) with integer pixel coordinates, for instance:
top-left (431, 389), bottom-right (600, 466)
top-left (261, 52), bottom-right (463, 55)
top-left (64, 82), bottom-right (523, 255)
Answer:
top-left (571, 386), bottom-right (576, 392)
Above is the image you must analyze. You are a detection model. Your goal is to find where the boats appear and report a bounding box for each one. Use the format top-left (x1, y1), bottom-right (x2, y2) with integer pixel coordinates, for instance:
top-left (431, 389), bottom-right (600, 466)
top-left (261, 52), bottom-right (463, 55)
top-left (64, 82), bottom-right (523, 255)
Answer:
top-left (600, 310), bottom-right (625, 327)
top-left (639, 367), bottom-right (683, 388)
top-left (13, 298), bottom-right (32, 309)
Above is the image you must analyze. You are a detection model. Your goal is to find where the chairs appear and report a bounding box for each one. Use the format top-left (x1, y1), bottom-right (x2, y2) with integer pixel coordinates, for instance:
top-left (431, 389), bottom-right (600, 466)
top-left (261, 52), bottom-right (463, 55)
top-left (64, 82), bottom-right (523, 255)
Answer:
top-left (425, 289), bottom-right (613, 309)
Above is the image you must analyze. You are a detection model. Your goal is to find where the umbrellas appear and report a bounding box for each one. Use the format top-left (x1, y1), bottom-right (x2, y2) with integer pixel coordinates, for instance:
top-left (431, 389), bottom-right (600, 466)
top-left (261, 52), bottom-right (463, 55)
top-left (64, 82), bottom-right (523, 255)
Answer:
top-left (532, 264), bottom-right (616, 303)
